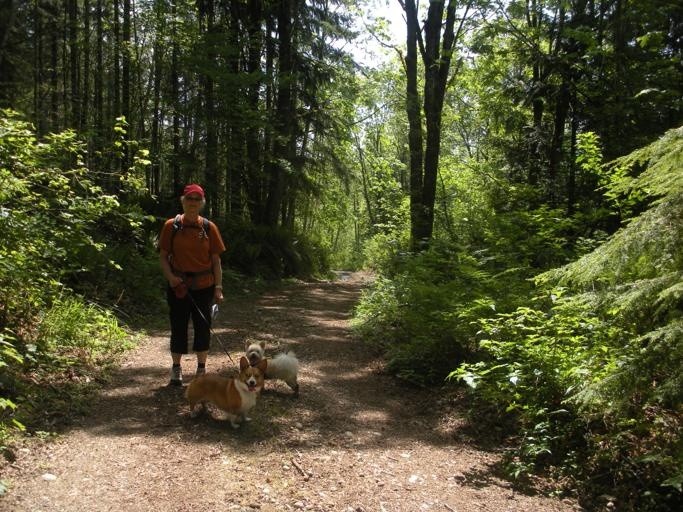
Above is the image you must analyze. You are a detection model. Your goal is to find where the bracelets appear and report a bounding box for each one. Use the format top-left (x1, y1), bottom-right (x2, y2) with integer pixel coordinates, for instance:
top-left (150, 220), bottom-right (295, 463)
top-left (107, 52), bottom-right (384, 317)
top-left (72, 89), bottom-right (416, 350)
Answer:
top-left (216, 286), bottom-right (224, 289)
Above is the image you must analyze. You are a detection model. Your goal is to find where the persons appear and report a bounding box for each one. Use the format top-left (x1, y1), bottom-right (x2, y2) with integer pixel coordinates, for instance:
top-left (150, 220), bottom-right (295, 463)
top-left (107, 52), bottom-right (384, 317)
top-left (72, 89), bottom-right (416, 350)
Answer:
top-left (158, 184), bottom-right (226, 385)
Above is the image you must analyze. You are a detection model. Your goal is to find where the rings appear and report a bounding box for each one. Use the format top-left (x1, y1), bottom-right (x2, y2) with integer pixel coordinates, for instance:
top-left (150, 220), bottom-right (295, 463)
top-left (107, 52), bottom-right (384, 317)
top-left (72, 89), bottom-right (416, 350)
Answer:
top-left (219, 296), bottom-right (223, 299)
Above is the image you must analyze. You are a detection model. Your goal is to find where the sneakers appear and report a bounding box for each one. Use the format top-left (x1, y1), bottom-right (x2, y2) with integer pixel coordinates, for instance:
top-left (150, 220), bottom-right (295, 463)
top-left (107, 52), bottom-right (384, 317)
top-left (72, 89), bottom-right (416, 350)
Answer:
top-left (170, 364), bottom-right (182, 384)
top-left (195, 366), bottom-right (205, 377)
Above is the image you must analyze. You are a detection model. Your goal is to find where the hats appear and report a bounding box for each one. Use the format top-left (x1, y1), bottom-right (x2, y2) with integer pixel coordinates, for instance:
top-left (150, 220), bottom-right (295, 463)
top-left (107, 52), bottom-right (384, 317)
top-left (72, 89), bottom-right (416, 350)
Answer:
top-left (182, 183), bottom-right (204, 197)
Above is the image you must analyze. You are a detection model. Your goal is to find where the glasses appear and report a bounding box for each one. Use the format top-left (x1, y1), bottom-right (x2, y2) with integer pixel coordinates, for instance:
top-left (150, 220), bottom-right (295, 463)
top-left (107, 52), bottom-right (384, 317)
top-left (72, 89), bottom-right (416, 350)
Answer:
top-left (185, 196), bottom-right (202, 201)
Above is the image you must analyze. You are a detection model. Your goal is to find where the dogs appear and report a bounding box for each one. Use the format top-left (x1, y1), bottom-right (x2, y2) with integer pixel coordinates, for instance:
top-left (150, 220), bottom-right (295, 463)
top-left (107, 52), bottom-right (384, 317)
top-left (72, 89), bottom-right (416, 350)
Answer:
top-left (184, 356), bottom-right (268, 431)
top-left (244, 338), bottom-right (301, 399)
